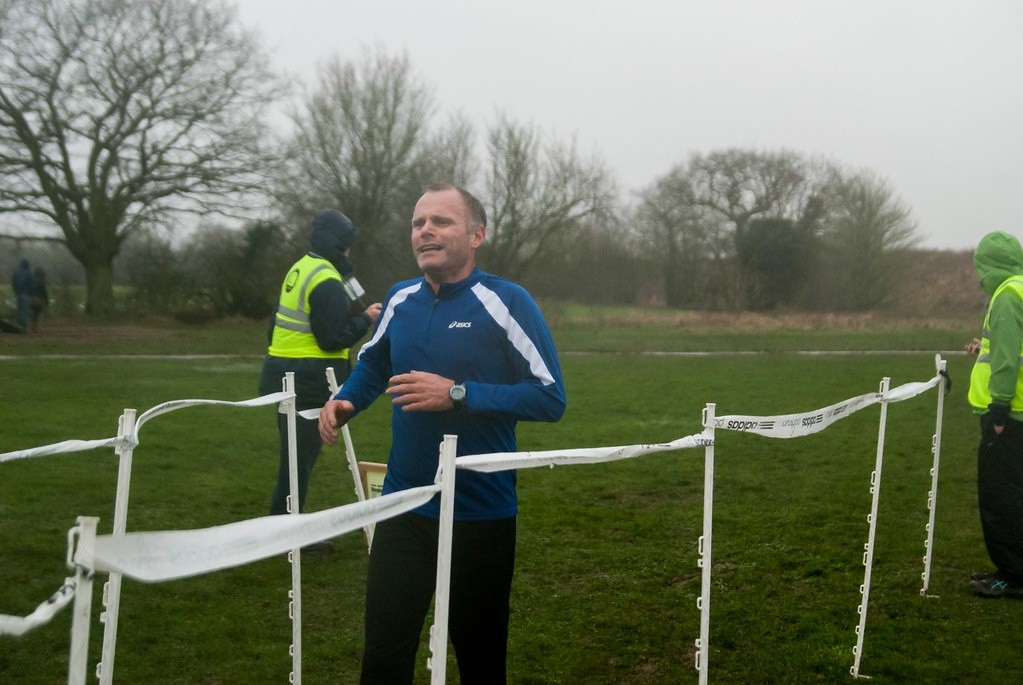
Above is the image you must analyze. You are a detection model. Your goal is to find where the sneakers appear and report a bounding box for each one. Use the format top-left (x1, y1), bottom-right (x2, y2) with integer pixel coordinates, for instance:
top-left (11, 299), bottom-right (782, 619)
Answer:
top-left (966, 571), bottom-right (1023, 600)
top-left (299, 541), bottom-right (334, 556)
top-left (970, 568), bottom-right (1001, 581)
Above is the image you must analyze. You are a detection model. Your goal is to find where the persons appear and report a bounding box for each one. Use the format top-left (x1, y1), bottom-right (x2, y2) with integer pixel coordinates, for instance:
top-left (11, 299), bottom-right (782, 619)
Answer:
top-left (12, 259), bottom-right (31, 329)
top-left (259, 208), bottom-right (383, 554)
top-left (964, 232), bottom-right (1023, 597)
top-left (318, 184), bottom-right (567, 685)
top-left (25, 265), bottom-right (49, 332)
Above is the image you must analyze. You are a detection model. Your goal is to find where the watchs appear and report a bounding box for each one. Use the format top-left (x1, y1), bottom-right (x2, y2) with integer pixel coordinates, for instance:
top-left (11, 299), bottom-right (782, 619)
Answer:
top-left (448, 376), bottom-right (468, 407)
